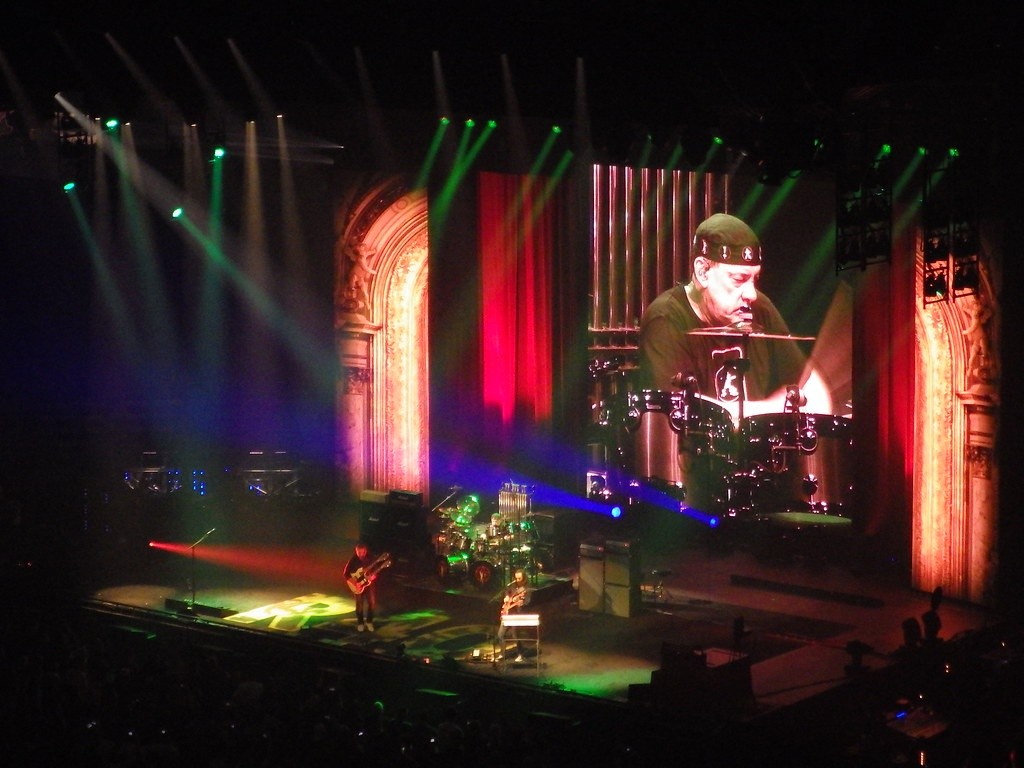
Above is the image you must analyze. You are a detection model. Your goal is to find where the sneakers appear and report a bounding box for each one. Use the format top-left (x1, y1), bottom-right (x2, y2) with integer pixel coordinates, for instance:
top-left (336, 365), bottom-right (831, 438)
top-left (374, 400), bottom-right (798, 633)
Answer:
top-left (514, 654), bottom-right (526, 662)
top-left (356, 622), bottom-right (364, 632)
top-left (494, 654), bottom-right (506, 662)
top-left (364, 621), bottom-right (374, 632)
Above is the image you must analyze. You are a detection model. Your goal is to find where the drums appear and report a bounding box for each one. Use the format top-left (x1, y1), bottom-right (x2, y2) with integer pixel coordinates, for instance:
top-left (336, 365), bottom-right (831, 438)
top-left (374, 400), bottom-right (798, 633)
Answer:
top-left (596, 390), bottom-right (731, 528)
top-left (451, 495), bottom-right (480, 524)
top-left (434, 521), bottom-right (538, 588)
top-left (732, 409), bottom-right (853, 516)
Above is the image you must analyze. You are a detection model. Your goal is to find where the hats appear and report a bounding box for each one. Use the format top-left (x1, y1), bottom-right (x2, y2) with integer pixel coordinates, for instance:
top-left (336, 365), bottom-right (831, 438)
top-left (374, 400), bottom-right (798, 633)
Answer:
top-left (692, 212), bottom-right (763, 266)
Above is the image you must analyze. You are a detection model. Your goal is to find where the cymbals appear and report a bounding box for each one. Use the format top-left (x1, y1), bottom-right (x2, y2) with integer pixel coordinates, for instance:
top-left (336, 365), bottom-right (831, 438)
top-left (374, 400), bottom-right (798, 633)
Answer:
top-left (439, 508), bottom-right (457, 515)
top-left (686, 320), bottom-right (815, 339)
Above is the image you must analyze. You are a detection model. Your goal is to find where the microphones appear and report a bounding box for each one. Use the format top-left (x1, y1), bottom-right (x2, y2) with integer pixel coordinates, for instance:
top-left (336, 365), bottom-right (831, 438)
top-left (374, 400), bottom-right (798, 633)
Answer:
top-left (207, 527), bottom-right (216, 535)
top-left (507, 580), bottom-right (516, 587)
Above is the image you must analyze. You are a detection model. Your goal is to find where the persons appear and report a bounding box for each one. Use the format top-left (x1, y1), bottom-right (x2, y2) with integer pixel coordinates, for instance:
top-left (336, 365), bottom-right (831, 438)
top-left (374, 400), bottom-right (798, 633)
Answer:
top-left (638, 212), bottom-right (833, 428)
top-left (344, 543), bottom-right (378, 632)
top-left (496, 569), bottom-right (529, 662)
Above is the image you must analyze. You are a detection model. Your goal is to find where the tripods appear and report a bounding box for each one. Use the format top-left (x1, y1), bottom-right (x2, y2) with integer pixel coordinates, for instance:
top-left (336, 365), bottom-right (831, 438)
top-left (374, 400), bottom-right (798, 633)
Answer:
top-left (487, 586), bottom-right (514, 675)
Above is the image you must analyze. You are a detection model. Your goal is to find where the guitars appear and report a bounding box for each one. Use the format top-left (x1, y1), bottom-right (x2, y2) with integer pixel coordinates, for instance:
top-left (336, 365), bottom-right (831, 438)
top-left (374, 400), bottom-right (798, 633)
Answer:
top-left (501, 588), bottom-right (526, 615)
top-left (347, 551), bottom-right (394, 594)
